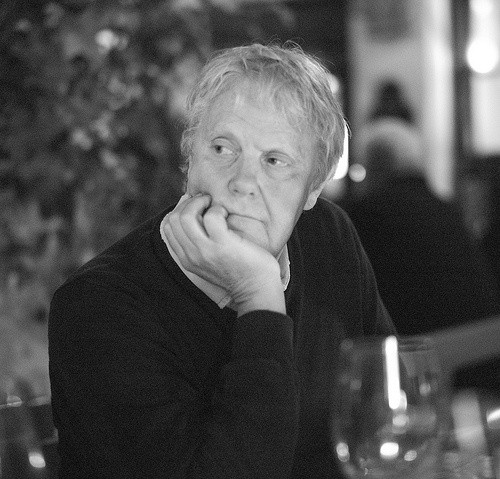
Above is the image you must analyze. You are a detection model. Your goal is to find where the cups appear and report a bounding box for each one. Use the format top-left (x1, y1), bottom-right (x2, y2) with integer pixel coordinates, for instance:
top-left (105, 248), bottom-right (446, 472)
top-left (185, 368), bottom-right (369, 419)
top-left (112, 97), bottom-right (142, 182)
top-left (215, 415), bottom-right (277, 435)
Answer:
top-left (331, 337), bottom-right (447, 477)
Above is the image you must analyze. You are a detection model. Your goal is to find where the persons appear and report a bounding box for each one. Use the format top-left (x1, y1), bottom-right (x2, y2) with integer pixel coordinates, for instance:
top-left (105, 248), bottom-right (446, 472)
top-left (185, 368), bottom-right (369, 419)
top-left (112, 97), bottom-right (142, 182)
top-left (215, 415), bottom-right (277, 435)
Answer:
top-left (47, 40), bottom-right (414, 479)
